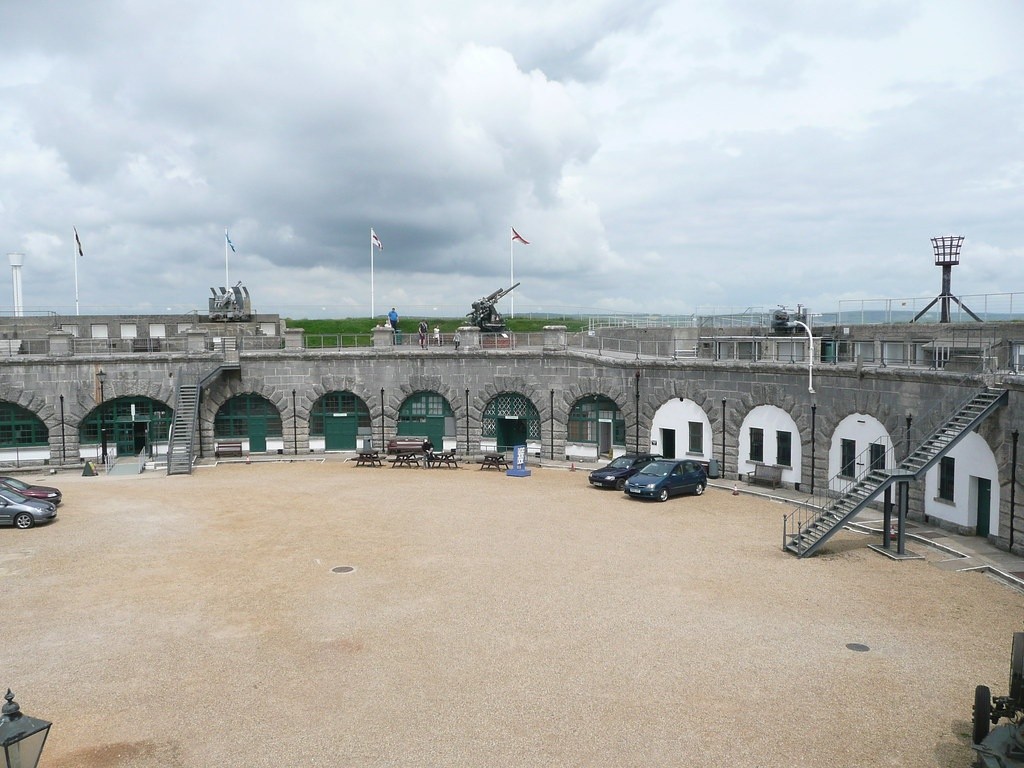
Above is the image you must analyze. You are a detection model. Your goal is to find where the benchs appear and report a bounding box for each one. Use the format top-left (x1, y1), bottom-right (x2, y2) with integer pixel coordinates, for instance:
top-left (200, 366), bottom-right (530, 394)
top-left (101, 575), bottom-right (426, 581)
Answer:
top-left (214, 440), bottom-right (242, 459)
top-left (388, 457), bottom-right (423, 463)
top-left (351, 456), bottom-right (387, 462)
top-left (426, 458), bottom-right (462, 463)
top-left (429, 334), bottom-right (454, 346)
top-left (476, 459), bottom-right (513, 465)
top-left (388, 439), bottom-right (425, 453)
top-left (131, 336), bottom-right (160, 352)
top-left (748, 464), bottom-right (783, 490)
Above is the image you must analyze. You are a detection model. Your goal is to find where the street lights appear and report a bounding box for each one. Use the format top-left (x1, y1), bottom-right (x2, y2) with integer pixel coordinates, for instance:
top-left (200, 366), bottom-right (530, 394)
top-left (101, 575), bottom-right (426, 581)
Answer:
top-left (96, 370), bottom-right (107, 464)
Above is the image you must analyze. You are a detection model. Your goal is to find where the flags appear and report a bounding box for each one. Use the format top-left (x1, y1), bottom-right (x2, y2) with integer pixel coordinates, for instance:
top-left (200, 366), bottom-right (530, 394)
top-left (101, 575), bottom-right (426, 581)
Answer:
top-left (372, 231), bottom-right (383, 250)
top-left (225, 233), bottom-right (235, 252)
top-left (512, 228), bottom-right (530, 245)
top-left (75, 229), bottom-right (83, 256)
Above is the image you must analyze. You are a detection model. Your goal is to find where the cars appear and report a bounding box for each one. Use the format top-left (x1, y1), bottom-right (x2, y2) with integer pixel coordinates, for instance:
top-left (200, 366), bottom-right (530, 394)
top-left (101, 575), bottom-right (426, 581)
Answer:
top-left (0, 477), bottom-right (62, 505)
top-left (0, 487), bottom-right (57, 529)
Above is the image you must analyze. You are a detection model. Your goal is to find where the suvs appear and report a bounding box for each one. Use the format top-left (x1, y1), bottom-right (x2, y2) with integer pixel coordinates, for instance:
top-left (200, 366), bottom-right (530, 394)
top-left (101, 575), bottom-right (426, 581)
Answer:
top-left (588, 454), bottom-right (665, 492)
top-left (624, 459), bottom-right (707, 502)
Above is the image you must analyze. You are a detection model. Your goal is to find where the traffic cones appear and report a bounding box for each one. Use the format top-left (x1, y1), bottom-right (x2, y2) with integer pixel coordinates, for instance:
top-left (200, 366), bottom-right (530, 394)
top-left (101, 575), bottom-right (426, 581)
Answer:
top-left (569, 463), bottom-right (576, 472)
top-left (732, 484), bottom-right (739, 495)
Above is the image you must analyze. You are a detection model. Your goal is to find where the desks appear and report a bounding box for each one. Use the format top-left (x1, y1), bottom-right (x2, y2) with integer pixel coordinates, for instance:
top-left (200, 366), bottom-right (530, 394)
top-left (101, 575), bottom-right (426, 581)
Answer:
top-left (480, 453), bottom-right (509, 471)
top-left (432, 452), bottom-right (458, 469)
top-left (355, 450), bottom-right (382, 468)
top-left (392, 452), bottom-right (420, 469)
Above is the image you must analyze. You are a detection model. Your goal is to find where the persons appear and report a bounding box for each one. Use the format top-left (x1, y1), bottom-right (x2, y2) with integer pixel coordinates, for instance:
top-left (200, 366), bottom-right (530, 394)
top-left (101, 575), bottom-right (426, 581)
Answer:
top-left (418, 320), bottom-right (428, 349)
top-left (388, 308), bottom-right (399, 334)
top-left (423, 438), bottom-right (434, 454)
top-left (434, 324), bottom-right (440, 346)
top-left (453, 330), bottom-right (460, 350)
